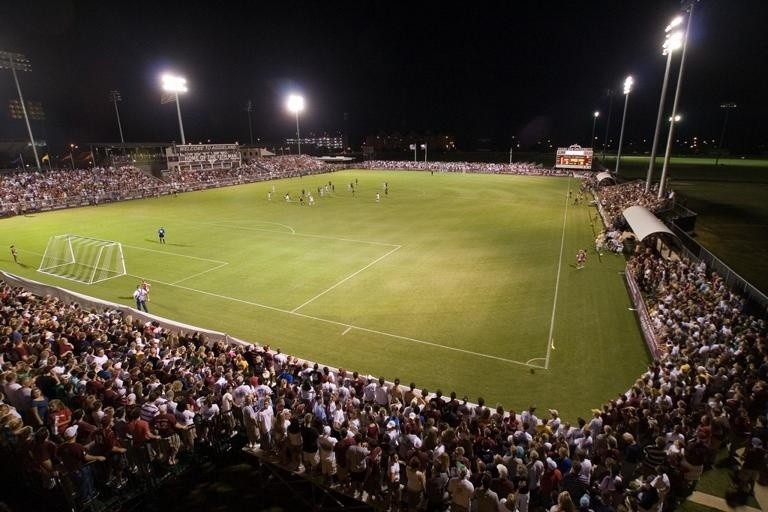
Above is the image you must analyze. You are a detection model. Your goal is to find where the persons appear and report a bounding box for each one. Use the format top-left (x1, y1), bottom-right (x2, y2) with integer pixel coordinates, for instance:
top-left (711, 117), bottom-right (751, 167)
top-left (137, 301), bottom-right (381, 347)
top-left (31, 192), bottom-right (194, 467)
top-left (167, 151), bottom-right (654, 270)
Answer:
top-left (1, 145), bottom-right (767, 512)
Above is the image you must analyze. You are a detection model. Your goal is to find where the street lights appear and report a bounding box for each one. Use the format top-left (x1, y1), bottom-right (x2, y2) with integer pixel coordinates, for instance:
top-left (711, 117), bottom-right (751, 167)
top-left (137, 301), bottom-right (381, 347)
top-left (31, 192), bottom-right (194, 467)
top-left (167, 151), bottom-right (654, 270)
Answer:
top-left (6, 99), bottom-right (47, 136)
top-left (613, 75), bottom-right (635, 174)
top-left (504, 136), bottom-right (520, 163)
top-left (591, 111), bottom-right (599, 150)
top-left (285, 96), bottom-right (308, 152)
top-left (602, 89), bottom-right (616, 160)
top-left (241, 99), bottom-right (257, 148)
top-left (106, 89), bottom-right (125, 143)
top-left (713, 101), bottom-right (738, 165)
top-left (160, 71), bottom-right (189, 147)
top-left (1, 51), bottom-right (47, 171)
